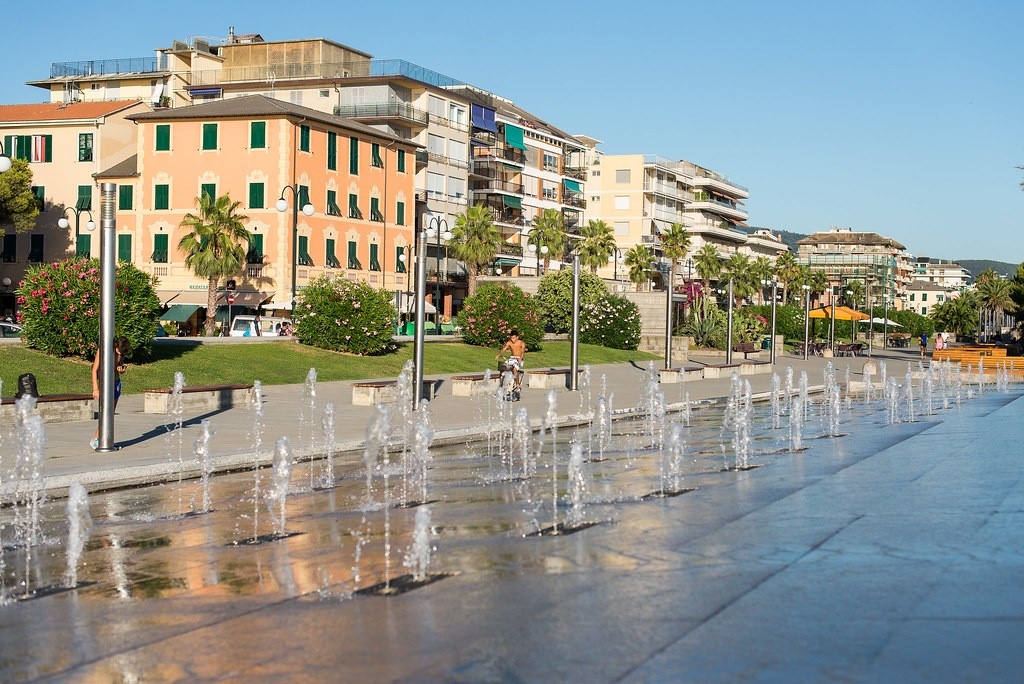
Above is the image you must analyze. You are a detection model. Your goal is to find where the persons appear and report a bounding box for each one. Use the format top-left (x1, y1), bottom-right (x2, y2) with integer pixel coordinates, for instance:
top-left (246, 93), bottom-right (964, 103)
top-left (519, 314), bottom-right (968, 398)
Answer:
top-left (941, 329), bottom-right (950, 349)
top-left (255, 315), bottom-right (263, 336)
top-left (919, 327), bottom-right (929, 360)
top-left (90, 335), bottom-right (131, 450)
top-left (494, 330), bottom-right (525, 398)
top-left (935, 333), bottom-right (944, 351)
top-left (990, 337), bottom-right (1016, 347)
top-left (895, 336), bottom-right (902, 347)
top-left (279, 322), bottom-right (289, 336)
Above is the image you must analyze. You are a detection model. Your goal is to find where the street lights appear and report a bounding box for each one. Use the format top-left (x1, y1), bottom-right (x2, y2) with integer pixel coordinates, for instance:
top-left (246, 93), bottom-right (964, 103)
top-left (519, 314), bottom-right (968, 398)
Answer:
top-left (399, 245), bottom-right (417, 321)
top-left (979, 307), bottom-right (1013, 343)
top-left (571, 257), bottom-right (580, 390)
top-left (726, 281), bottom-right (733, 364)
top-left (528, 235), bottom-right (548, 276)
top-left (608, 248), bottom-right (622, 280)
top-left (884, 304), bottom-right (887, 350)
top-left (96, 182), bottom-right (121, 452)
top-left (57, 207), bottom-right (96, 257)
top-left (831, 295), bottom-right (834, 357)
top-left (276, 185), bottom-right (315, 324)
top-left (804, 290), bottom-right (810, 360)
top-left (666, 271), bottom-right (671, 370)
top-left (761, 276), bottom-right (771, 306)
top-left (412, 233), bottom-right (427, 412)
top-left (427, 216), bottom-right (452, 335)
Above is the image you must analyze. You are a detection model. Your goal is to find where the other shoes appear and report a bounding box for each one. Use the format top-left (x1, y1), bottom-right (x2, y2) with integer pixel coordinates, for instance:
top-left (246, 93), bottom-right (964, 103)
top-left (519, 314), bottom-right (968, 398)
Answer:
top-left (90, 439), bottom-right (99, 450)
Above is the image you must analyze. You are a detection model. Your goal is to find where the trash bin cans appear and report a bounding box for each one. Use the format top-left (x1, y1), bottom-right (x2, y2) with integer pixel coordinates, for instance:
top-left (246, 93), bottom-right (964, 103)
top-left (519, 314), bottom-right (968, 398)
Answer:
top-left (763, 337), bottom-right (772, 349)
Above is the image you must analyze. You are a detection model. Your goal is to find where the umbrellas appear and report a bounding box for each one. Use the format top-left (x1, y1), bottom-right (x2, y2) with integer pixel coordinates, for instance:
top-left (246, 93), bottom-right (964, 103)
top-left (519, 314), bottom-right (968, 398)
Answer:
top-left (803, 304), bottom-right (904, 350)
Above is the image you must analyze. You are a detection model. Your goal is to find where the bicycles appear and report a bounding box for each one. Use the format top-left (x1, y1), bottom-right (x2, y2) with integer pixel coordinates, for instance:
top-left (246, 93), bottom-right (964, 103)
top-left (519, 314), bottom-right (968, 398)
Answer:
top-left (497, 355), bottom-right (524, 402)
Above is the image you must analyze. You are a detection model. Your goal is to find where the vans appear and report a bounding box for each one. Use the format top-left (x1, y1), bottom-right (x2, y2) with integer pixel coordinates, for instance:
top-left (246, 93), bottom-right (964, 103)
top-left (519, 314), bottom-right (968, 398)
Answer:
top-left (229, 315), bottom-right (293, 337)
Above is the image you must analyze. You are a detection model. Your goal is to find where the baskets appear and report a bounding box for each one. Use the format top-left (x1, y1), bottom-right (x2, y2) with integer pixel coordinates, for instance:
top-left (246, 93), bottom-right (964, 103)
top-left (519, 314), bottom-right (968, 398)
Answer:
top-left (498, 362), bottom-right (508, 371)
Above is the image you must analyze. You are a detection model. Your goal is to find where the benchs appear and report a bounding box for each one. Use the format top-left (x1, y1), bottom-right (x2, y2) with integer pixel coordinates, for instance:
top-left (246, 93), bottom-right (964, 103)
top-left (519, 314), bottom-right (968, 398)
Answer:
top-left (659, 367), bottom-right (703, 383)
top-left (451, 373), bottom-right (501, 397)
top-left (737, 342), bottom-right (762, 353)
top-left (0, 393), bottom-right (95, 424)
top-left (740, 361), bottom-right (772, 375)
top-left (703, 361), bottom-right (741, 378)
top-left (528, 368), bottom-right (584, 388)
top-left (142, 383), bottom-right (253, 414)
top-left (933, 347), bottom-right (1024, 377)
top-left (349, 379), bottom-right (438, 406)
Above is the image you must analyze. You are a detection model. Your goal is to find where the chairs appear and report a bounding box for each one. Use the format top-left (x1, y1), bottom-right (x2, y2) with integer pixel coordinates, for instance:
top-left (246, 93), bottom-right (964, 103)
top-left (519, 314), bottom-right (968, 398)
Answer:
top-left (794, 341), bottom-right (864, 357)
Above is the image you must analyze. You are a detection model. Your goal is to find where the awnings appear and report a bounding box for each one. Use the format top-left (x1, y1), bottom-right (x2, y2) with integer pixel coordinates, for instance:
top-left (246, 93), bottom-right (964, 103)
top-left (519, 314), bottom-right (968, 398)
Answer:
top-left (167, 289), bottom-right (225, 309)
top-left (160, 305), bottom-right (200, 322)
top-left (215, 290), bottom-right (275, 310)
top-left (154, 289), bottom-right (181, 308)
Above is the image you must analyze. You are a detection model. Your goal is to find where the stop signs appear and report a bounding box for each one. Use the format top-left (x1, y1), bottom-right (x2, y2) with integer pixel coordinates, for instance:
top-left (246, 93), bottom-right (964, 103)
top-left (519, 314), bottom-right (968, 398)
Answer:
top-left (228, 295), bottom-right (234, 304)
